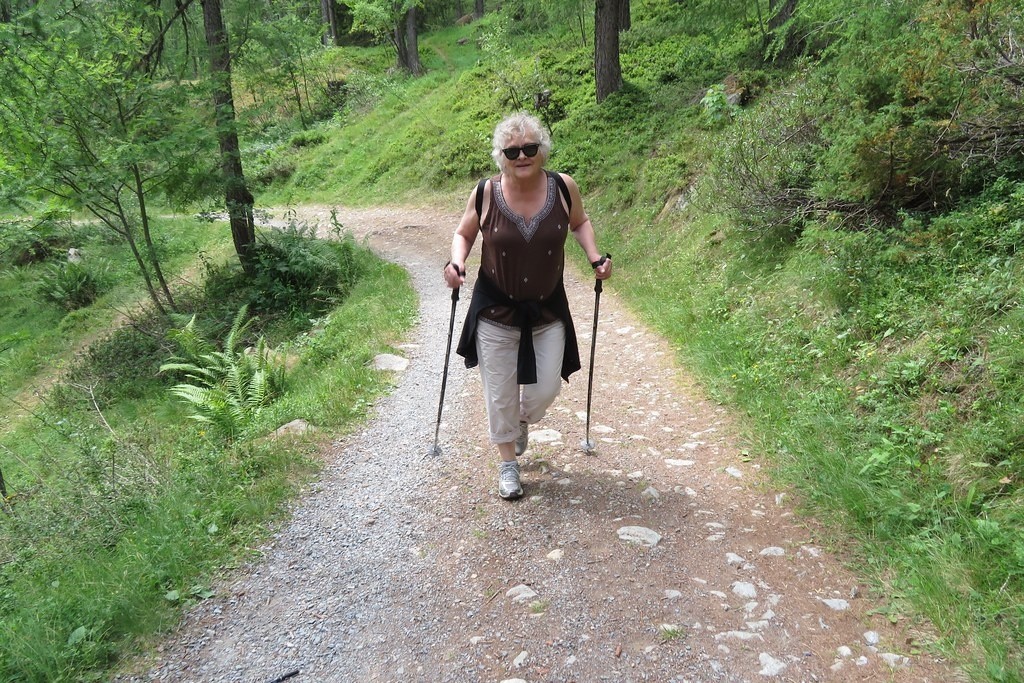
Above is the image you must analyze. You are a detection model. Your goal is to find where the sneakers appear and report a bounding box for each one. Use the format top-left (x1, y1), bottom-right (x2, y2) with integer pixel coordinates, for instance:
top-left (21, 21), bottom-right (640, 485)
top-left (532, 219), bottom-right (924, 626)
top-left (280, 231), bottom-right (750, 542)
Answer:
top-left (514, 420), bottom-right (528, 455)
top-left (498, 461), bottom-right (524, 498)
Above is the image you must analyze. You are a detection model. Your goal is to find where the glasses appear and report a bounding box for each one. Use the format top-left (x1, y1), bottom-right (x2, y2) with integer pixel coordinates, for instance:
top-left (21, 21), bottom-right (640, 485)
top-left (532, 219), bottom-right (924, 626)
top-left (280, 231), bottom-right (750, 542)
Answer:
top-left (502, 143), bottom-right (540, 160)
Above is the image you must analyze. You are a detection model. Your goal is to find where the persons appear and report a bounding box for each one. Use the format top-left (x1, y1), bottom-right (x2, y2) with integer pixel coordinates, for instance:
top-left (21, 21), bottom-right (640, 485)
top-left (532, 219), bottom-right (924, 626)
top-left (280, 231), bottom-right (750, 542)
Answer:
top-left (444, 109), bottom-right (613, 501)
